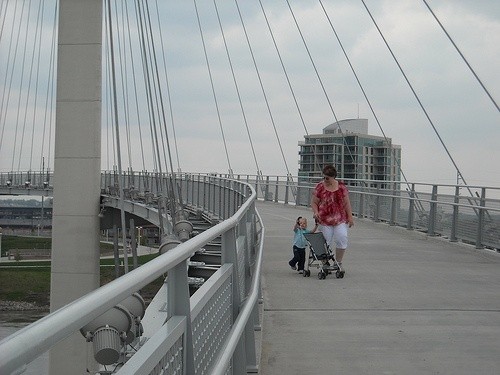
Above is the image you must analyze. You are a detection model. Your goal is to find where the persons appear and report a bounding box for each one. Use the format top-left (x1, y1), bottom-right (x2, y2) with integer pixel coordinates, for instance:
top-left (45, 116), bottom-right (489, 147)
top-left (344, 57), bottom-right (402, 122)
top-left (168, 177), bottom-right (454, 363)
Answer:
top-left (289, 217), bottom-right (319, 274)
top-left (313, 165), bottom-right (354, 273)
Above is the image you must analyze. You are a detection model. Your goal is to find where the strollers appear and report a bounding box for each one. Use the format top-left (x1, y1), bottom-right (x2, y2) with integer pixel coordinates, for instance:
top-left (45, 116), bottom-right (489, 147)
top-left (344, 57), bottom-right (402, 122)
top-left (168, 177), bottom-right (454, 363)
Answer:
top-left (297, 217), bottom-right (345, 281)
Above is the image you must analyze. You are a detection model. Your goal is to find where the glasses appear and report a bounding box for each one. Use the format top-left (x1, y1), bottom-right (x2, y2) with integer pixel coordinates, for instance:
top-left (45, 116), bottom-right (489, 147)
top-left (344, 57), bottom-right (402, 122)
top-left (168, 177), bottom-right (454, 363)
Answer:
top-left (322, 176), bottom-right (333, 180)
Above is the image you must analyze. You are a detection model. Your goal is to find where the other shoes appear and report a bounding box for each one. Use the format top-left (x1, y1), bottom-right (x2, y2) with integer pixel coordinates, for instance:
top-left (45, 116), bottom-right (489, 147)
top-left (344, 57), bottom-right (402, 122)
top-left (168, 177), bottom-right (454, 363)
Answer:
top-left (298, 270), bottom-right (304, 274)
top-left (333, 262), bottom-right (345, 273)
top-left (291, 266), bottom-right (296, 270)
